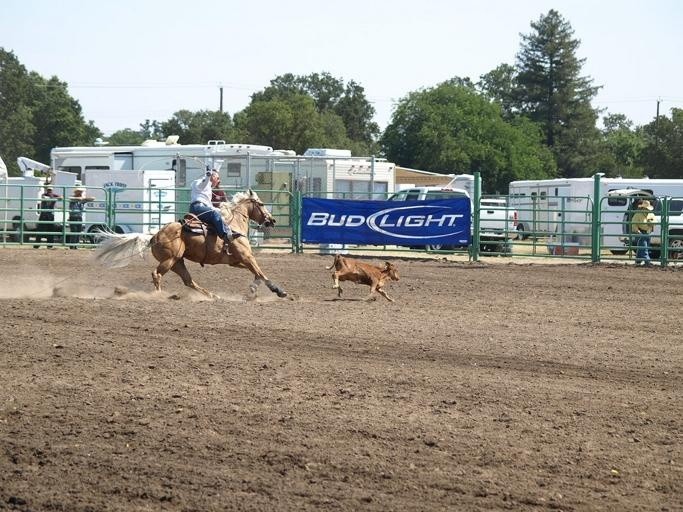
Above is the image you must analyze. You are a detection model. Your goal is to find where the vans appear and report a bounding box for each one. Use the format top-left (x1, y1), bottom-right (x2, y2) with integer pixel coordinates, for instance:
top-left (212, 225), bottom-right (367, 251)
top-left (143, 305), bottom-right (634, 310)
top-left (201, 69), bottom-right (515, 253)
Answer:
top-left (604, 187), bottom-right (682, 261)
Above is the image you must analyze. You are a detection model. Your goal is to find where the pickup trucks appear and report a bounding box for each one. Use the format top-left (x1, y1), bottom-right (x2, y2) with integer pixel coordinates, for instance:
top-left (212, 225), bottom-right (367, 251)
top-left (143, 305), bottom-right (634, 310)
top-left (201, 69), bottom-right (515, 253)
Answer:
top-left (385, 186), bottom-right (519, 258)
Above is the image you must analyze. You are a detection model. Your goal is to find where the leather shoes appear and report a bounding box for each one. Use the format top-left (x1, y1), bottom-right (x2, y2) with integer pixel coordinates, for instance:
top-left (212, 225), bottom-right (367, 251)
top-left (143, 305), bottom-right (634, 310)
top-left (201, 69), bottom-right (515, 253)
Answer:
top-left (223, 232), bottom-right (241, 242)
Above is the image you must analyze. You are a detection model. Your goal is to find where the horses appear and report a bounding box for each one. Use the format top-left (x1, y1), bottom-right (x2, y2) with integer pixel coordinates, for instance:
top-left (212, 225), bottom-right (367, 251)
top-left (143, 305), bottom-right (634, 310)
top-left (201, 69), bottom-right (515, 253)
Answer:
top-left (92, 187), bottom-right (288, 299)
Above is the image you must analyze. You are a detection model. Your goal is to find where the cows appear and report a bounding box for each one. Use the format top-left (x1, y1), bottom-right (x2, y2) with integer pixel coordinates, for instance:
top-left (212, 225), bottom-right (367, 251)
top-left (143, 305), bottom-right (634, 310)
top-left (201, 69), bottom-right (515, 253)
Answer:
top-left (325, 252), bottom-right (401, 302)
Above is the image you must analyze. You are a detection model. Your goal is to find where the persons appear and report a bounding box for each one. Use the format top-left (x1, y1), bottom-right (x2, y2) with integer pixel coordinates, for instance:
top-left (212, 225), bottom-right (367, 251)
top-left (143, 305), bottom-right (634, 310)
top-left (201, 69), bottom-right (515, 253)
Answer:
top-left (65, 185), bottom-right (94, 249)
top-left (211, 181), bottom-right (226, 208)
top-left (631, 200), bottom-right (654, 267)
top-left (184, 168), bottom-right (241, 245)
top-left (34, 187), bottom-right (62, 249)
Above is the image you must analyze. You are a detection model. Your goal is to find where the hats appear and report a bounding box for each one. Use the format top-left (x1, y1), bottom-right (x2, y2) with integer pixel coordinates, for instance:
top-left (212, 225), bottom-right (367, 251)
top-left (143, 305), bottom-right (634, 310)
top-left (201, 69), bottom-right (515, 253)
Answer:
top-left (72, 187), bottom-right (85, 192)
